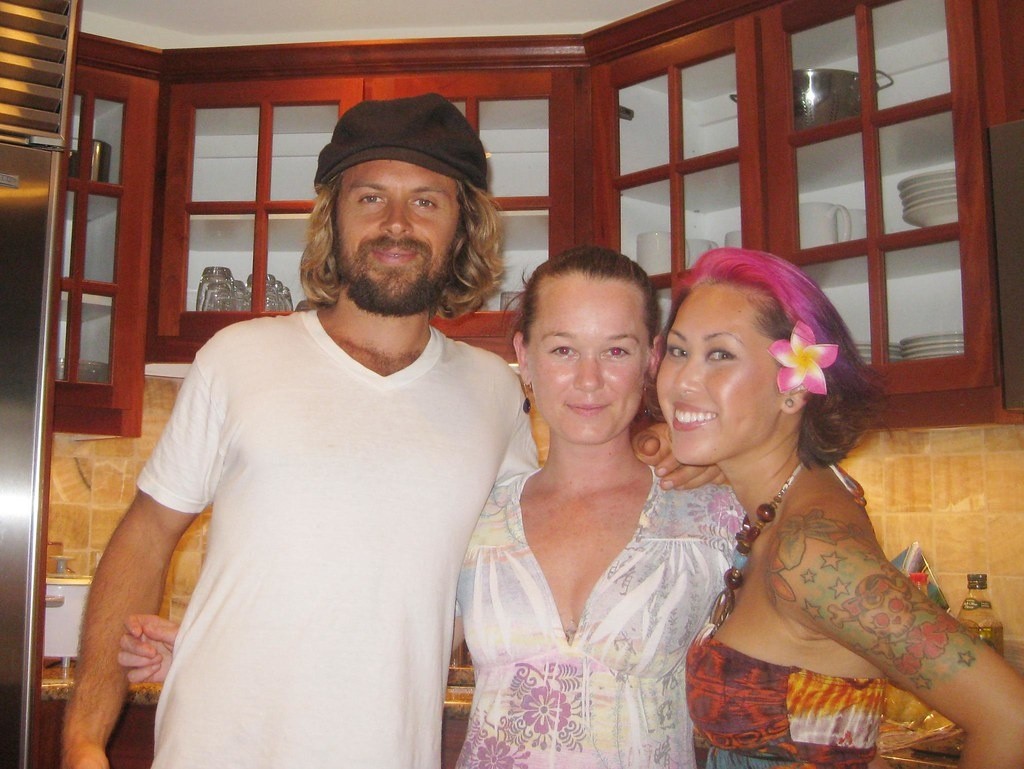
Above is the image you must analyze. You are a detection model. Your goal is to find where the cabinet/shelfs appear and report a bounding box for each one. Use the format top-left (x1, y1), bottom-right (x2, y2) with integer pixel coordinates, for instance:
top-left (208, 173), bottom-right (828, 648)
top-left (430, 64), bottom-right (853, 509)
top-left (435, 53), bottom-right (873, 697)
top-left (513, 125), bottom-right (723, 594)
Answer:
top-left (51, 0), bottom-right (1024, 437)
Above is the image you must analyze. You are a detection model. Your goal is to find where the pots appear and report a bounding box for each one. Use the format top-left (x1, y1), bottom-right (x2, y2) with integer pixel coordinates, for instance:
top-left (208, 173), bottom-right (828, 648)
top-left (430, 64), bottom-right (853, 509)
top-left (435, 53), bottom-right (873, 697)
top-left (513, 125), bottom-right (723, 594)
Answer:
top-left (729, 68), bottom-right (894, 130)
top-left (44, 554), bottom-right (95, 672)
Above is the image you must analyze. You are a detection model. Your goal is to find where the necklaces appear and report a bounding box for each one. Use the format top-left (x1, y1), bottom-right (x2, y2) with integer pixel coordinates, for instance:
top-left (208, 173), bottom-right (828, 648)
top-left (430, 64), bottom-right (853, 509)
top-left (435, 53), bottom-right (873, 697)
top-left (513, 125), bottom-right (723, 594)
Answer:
top-left (724, 458), bottom-right (806, 589)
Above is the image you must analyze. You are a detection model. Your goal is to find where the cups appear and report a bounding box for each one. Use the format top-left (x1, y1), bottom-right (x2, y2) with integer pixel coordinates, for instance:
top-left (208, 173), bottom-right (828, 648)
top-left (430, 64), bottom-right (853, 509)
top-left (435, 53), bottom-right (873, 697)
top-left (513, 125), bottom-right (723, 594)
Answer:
top-left (636, 231), bottom-right (689, 276)
top-left (687, 239), bottom-right (718, 269)
top-left (500, 292), bottom-right (522, 311)
top-left (724, 231), bottom-right (741, 248)
top-left (195, 267), bottom-right (293, 313)
top-left (799, 203), bottom-right (850, 252)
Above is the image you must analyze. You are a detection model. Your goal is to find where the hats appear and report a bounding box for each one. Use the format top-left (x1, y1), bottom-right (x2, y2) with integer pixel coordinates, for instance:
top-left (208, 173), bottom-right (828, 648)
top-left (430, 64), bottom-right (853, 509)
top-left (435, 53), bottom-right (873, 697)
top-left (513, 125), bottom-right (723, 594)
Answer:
top-left (313, 92), bottom-right (488, 195)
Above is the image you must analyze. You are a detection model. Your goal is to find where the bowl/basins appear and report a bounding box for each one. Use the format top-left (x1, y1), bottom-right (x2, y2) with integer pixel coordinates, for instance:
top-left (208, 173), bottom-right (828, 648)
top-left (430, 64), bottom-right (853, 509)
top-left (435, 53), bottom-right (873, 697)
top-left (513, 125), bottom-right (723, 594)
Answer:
top-left (57, 358), bottom-right (110, 383)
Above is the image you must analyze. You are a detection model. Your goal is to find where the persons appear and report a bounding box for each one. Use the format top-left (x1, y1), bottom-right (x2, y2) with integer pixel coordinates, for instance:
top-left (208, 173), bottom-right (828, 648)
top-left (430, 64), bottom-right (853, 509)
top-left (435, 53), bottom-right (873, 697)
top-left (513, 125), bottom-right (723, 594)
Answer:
top-left (60, 93), bottom-right (725, 769)
top-left (117, 246), bottom-right (745, 769)
top-left (653, 247), bottom-right (1024, 769)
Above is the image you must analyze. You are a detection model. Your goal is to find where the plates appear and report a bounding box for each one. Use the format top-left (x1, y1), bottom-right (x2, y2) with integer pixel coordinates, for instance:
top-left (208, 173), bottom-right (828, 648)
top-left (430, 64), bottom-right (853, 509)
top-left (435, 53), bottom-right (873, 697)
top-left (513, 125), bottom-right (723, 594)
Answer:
top-left (897, 169), bottom-right (959, 227)
top-left (854, 332), bottom-right (964, 364)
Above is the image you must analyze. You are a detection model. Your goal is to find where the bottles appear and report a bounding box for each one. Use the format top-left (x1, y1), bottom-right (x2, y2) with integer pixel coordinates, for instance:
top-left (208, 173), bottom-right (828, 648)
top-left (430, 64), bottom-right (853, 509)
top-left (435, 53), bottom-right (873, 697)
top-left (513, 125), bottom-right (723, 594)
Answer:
top-left (45, 540), bottom-right (74, 578)
top-left (883, 573), bottom-right (928, 730)
top-left (957, 574), bottom-right (1004, 660)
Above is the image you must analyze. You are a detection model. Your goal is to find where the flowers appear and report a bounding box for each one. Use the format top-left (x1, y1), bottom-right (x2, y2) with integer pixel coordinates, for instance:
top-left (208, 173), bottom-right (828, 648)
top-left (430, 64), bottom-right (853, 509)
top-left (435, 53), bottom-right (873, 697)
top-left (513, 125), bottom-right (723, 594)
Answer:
top-left (771, 320), bottom-right (839, 395)
